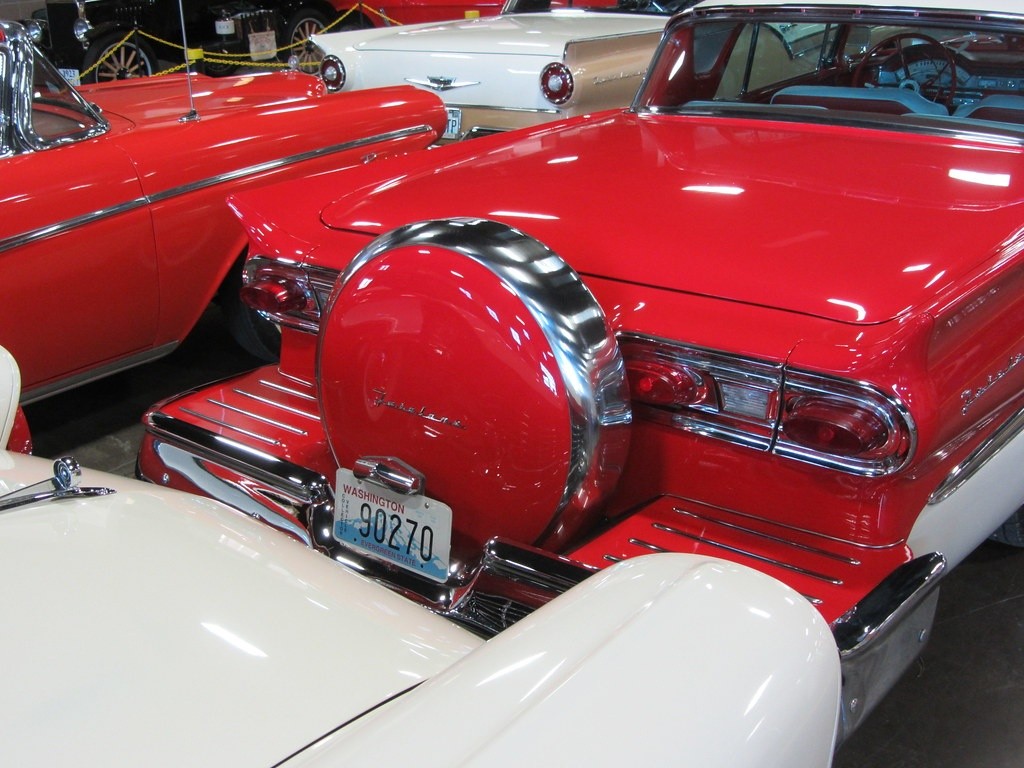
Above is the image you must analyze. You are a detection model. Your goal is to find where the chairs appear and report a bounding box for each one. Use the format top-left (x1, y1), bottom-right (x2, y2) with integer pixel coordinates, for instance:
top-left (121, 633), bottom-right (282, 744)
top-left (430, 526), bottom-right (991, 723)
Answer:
top-left (683, 85), bottom-right (1024, 133)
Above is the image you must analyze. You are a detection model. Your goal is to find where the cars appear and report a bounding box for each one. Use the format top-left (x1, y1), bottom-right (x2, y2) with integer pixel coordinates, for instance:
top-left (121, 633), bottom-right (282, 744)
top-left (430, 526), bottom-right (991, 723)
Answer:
top-left (0, 1), bottom-right (632, 91)
top-left (154, 1), bottom-right (1023, 748)
top-left (1, 1), bottom-right (454, 458)
top-left (290, 2), bottom-right (914, 142)
top-left (0, 469), bottom-right (839, 768)
